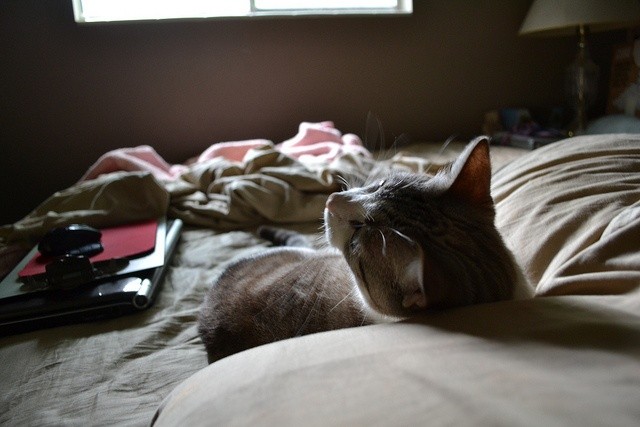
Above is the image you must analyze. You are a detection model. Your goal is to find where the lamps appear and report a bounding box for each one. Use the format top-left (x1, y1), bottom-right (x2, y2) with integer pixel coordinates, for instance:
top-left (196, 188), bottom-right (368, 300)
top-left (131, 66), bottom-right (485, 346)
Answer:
top-left (517, 1), bottom-right (639, 134)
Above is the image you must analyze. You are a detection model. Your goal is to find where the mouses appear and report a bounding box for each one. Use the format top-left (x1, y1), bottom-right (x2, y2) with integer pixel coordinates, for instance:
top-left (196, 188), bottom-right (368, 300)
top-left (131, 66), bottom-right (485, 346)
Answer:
top-left (37, 222), bottom-right (102, 259)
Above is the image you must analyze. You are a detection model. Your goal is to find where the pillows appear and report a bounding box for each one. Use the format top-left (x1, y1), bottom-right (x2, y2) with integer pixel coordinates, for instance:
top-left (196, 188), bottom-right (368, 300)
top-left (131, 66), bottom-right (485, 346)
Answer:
top-left (147, 293), bottom-right (639, 427)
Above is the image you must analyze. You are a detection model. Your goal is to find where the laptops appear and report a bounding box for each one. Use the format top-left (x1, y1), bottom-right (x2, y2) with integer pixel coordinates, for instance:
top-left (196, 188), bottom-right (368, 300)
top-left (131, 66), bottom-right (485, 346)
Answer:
top-left (0, 217), bottom-right (185, 338)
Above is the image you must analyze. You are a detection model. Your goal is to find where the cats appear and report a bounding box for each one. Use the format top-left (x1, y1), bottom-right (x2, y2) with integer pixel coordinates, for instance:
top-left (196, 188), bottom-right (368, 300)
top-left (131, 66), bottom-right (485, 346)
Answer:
top-left (197, 136), bottom-right (532, 364)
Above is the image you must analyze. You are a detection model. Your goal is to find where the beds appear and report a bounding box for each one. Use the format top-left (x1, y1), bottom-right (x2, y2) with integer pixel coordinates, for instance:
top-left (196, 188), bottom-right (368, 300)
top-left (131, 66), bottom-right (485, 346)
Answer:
top-left (0, 140), bottom-right (639, 427)
